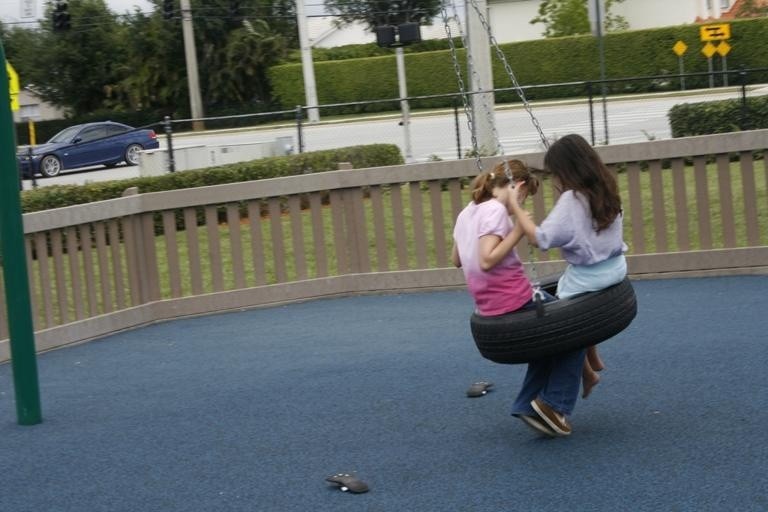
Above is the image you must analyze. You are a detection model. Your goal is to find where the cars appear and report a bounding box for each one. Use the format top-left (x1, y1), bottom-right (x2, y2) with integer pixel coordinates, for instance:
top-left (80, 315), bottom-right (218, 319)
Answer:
top-left (15, 120), bottom-right (160, 179)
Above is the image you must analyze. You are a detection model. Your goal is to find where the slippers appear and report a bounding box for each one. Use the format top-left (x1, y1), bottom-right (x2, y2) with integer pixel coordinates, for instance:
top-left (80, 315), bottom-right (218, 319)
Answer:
top-left (465, 376), bottom-right (494, 397)
top-left (324, 472), bottom-right (369, 494)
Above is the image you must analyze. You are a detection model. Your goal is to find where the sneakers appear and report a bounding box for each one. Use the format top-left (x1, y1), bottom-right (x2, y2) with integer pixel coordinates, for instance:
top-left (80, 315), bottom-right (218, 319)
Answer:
top-left (529, 397), bottom-right (572, 436)
top-left (520, 412), bottom-right (557, 437)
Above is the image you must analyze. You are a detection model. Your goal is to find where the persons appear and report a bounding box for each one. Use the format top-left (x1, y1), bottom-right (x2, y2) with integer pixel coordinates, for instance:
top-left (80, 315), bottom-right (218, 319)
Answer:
top-left (502, 133), bottom-right (627, 399)
top-left (447, 153), bottom-right (580, 441)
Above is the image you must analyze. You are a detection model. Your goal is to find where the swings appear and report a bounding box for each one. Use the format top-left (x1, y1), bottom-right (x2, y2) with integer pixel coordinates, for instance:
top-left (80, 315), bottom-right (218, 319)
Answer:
top-left (438, 0), bottom-right (637, 364)
